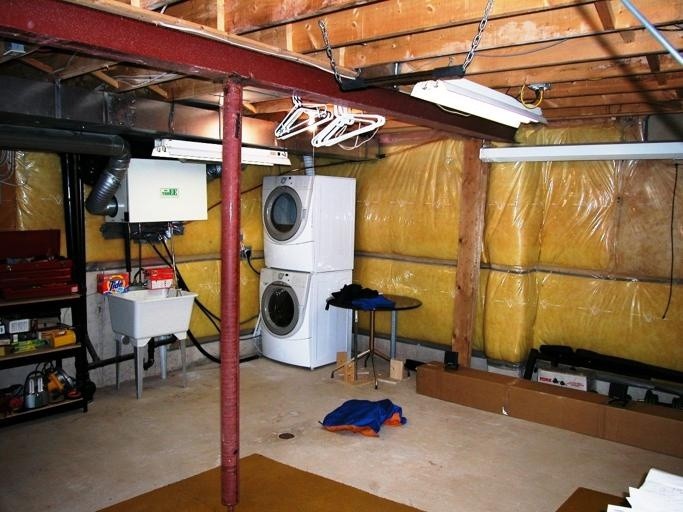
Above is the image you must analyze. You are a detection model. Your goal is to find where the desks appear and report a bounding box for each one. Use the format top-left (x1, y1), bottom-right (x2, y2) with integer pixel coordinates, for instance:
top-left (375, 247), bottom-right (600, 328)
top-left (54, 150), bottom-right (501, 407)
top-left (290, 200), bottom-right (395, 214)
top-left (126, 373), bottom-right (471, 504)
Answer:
top-left (325, 296), bottom-right (423, 388)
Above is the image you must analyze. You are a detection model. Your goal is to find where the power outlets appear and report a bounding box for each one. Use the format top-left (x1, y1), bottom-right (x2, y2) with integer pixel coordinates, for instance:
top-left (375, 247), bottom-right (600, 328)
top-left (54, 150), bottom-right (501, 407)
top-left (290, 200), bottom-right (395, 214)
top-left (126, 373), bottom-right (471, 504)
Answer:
top-left (241, 247), bottom-right (251, 259)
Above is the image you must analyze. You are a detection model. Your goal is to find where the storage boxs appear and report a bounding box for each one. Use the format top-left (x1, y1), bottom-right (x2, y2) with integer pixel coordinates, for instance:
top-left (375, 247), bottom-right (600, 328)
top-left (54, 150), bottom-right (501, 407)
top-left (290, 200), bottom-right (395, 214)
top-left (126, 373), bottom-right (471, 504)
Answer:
top-left (505, 379), bottom-right (613, 439)
top-left (0, 228), bottom-right (72, 291)
top-left (606, 399), bottom-right (683, 455)
top-left (416, 359), bottom-right (517, 414)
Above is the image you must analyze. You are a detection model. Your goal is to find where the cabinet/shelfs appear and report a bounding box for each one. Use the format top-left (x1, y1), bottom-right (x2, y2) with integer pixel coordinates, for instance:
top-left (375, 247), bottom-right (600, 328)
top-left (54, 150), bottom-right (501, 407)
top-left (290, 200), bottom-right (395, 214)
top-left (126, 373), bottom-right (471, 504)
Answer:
top-left (0, 292), bottom-right (88, 428)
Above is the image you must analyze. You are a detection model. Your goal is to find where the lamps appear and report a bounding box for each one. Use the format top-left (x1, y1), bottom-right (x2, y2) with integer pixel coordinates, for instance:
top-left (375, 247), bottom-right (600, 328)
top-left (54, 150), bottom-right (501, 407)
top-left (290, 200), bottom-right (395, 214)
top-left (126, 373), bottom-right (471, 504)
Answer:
top-left (152, 136), bottom-right (290, 168)
top-left (475, 143), bottom-right (683, 161)
top-left (411, 77), bottom-right (548, 131)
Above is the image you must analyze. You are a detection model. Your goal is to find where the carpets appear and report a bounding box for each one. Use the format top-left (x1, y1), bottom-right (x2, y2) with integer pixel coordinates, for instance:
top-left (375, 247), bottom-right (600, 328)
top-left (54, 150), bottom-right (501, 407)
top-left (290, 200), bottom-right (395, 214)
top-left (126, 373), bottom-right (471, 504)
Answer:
top-left (94, 451), bottom-right (423, 512)
top-left (553, 486), bottom-right (629, 511)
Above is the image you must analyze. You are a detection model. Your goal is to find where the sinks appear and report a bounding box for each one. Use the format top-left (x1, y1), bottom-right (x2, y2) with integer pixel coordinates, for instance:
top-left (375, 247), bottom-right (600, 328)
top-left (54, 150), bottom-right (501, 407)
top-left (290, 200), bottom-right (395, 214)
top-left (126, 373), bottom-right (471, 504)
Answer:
top-left (107, 287), bottom-right (198, 348)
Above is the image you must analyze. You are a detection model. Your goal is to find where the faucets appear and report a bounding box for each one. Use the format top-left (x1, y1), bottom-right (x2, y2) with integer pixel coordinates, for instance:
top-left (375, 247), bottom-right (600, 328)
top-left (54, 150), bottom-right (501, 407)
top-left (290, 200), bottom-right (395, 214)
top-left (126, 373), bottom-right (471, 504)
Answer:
top-left (133, 268), bottom-right (145, 284)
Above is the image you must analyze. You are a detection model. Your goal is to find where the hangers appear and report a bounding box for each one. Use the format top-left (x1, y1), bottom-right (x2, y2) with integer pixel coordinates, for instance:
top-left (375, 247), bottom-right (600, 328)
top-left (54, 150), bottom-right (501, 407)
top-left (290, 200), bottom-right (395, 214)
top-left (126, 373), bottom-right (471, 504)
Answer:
top-left (275, 94), bottom-right (386, 149)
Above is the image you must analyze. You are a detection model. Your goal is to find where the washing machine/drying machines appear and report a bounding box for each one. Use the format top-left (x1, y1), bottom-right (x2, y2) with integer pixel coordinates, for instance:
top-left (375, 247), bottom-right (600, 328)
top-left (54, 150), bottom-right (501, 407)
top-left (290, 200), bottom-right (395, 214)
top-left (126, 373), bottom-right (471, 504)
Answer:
top-left (261, 174), bottom-right (357, 272)
top-left (258, 269), bottom-right (353, 370)
